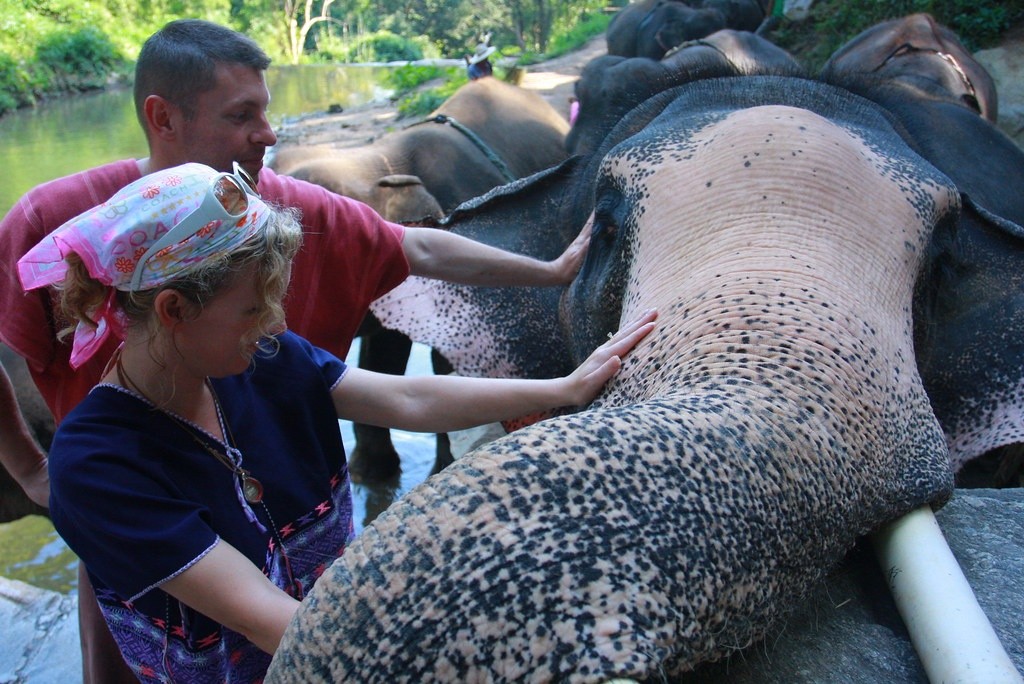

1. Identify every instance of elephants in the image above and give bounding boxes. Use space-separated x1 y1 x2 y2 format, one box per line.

263 1 1024 683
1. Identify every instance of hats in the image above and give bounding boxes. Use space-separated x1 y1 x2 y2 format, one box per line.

469 44 496 65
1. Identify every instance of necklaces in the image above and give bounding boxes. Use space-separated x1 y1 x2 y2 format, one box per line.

117 351 263 502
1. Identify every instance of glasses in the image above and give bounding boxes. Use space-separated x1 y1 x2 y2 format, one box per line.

130 160 262 291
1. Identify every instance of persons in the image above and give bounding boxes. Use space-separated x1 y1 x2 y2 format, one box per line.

467 44 496 80
17 162 657 684
0 18 597 684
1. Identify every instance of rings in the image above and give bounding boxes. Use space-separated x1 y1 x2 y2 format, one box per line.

607 332 614 339
